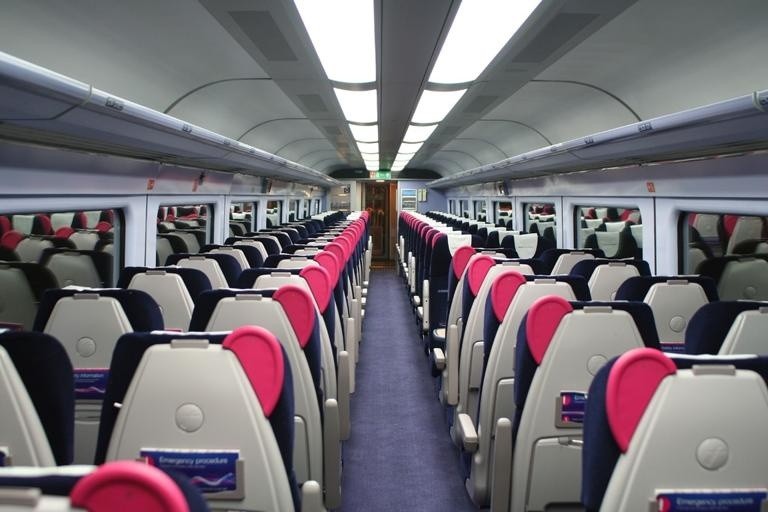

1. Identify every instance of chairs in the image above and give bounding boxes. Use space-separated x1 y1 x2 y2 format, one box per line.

0 205 373 512
395 203 768 511
